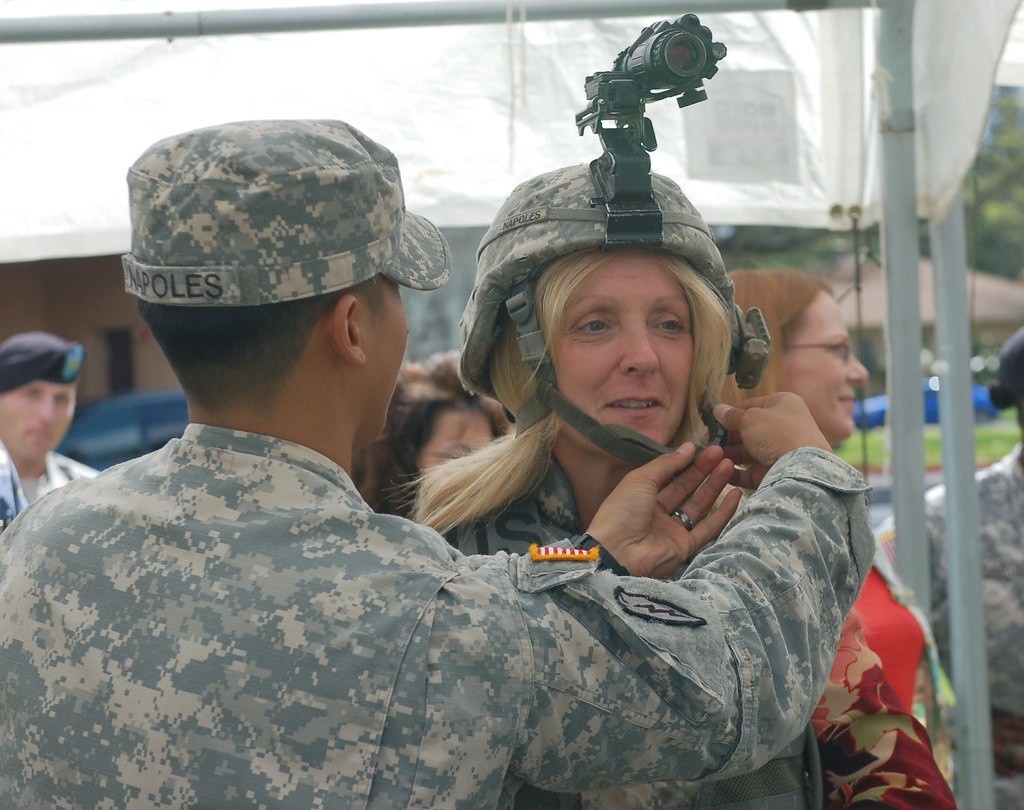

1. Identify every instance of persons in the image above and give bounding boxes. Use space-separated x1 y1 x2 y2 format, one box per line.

0 117 1023 810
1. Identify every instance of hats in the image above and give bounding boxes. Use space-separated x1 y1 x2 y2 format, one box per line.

121 119 451 306
989 328 1023 409
0 331 87 394
458 152 773 397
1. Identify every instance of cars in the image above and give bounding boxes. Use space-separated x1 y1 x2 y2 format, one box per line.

47 386 187 471
854 376 999 432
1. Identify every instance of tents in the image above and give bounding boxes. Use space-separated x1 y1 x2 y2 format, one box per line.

0 0 1024 807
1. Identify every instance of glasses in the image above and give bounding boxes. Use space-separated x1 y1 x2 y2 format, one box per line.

784 340 852 362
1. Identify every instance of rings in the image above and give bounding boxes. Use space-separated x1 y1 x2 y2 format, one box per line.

671 509 693 533
